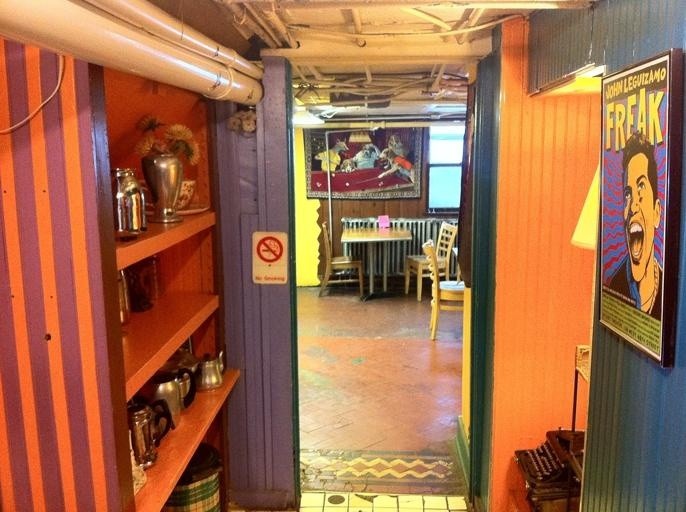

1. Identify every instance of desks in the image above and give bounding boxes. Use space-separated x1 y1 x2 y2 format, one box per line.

340 227 412 302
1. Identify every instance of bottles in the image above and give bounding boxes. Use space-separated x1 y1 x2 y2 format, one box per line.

117 269 131 327
107 166 147 242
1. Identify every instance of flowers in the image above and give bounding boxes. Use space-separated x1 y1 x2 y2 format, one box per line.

132 113 202 166
225 110 256 140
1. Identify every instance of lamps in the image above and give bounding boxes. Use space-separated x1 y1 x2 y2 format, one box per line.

569 162 602 252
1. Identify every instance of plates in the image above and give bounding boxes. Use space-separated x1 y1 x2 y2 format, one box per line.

141 205 211 216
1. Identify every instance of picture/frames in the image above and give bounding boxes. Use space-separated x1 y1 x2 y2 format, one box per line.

302 128 423 199
598 47 685 370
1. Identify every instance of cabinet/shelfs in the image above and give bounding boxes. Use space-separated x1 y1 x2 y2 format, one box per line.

114 208 242 512
545 345 592 512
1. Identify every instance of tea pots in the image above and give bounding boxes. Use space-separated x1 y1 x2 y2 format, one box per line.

126 349 225 499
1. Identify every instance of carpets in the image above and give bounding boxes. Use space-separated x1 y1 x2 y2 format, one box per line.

298 449 469 496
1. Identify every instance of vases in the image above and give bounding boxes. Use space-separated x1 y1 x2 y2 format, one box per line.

141 153 184 223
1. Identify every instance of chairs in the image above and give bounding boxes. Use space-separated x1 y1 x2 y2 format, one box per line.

319 222 364 301
405 221 458 302
420 239 465 341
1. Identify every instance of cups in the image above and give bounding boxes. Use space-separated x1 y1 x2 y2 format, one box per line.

138 179 196 210
140 252 166 300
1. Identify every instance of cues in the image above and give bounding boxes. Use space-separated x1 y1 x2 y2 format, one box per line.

356 177 378 184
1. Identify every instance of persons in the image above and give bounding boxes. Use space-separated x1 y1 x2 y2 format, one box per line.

605 131 663 320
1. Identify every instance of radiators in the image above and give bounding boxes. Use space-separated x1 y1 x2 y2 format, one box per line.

330 218 458 278
